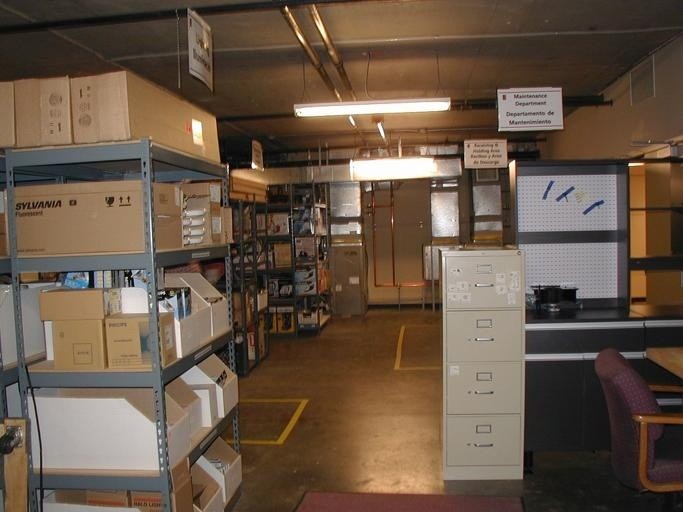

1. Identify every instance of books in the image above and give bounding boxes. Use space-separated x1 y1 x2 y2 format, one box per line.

60 267 192 321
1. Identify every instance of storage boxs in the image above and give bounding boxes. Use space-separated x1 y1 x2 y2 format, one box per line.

70 72 222 164
14 76 73 146
0 80 15 148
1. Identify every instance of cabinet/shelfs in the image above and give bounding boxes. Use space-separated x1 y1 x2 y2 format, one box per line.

438 242 533 482
528 328 647 451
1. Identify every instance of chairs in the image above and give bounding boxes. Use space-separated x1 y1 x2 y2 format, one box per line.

595 348 679 511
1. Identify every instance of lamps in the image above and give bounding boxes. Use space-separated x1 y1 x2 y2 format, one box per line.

292 44 451 119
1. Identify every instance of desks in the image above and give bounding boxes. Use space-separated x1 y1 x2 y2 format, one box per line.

640 346 682 380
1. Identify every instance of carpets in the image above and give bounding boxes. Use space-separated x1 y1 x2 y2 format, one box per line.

294 487 529 512
